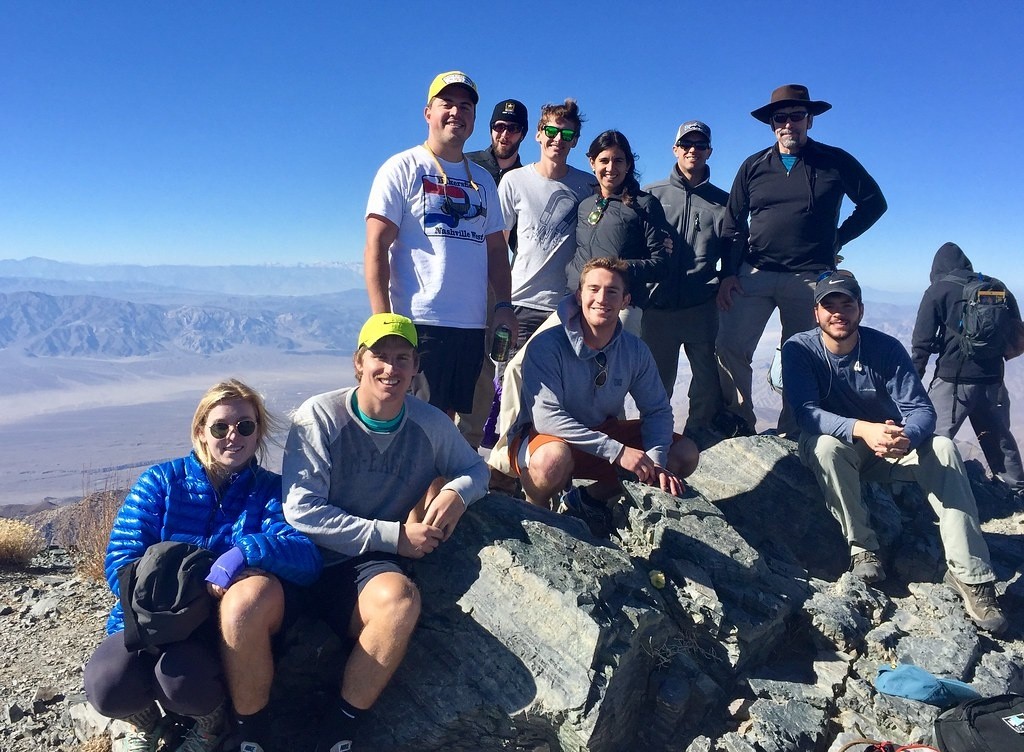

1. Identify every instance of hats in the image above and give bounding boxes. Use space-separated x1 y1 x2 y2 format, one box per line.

491 98 530 127
751 84 833 124
812 272 863 302
675 120 712 147
428 71 480 103
359 312 421 348
874 662 983 710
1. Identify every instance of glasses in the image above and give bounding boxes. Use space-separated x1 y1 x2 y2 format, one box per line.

813 268 855 282
541 126 577 143
587 198 610 226
677 140 709 150
595 350 609 387
770 111 812 123
492 123 525 134
207 419 259 440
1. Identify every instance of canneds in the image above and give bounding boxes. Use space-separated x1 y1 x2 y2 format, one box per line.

490 327 511 362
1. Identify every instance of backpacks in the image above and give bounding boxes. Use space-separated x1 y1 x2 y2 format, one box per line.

940 273 1014 361
938 689 1024 752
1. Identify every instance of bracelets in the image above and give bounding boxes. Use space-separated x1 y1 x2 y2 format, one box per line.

495 302 516 311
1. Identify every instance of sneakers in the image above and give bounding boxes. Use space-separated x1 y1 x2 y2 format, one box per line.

850 550 887 583
941 569 1009 635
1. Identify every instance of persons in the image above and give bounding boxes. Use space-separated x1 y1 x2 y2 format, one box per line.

697 85 888 447
364 71 518 435
216 312 492 752
642 117 738 448
452 99 528 453
83 377 321 752
487 256 700 519
912 243 1024 500
564 129 669 338
779 270 1008 633
496 97 672 348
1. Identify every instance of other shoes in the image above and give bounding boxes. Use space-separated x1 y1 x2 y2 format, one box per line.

314 734 353 752
178 717 231 752
122 716 166 751
225 735 265 752
